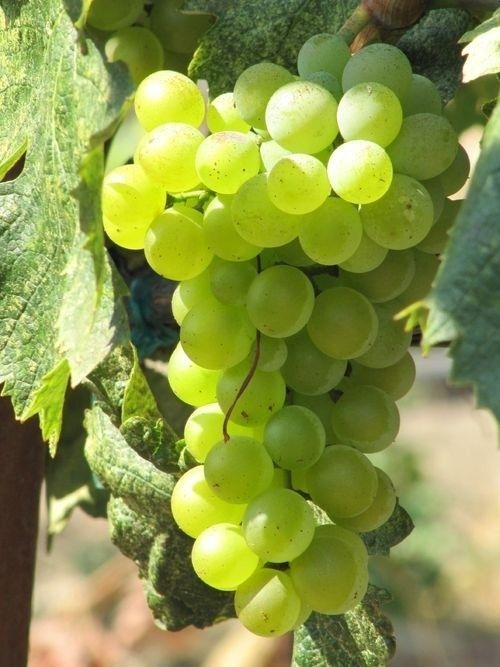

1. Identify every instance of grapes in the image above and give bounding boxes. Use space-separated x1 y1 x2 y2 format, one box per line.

100 33 469 637
86 0 216 91
